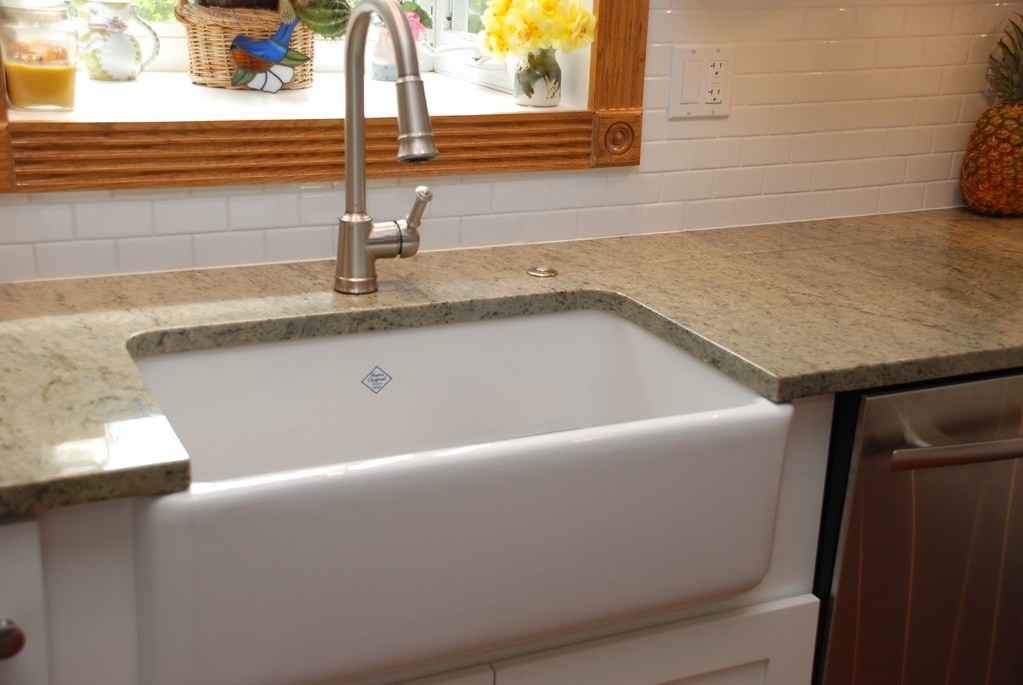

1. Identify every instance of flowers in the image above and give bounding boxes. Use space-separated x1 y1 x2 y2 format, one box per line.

382 0 432 47
482 0 597 56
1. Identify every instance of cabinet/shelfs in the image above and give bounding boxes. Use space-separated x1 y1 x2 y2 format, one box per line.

815 372 1023 685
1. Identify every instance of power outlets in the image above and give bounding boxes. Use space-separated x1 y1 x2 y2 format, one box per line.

669 44 735 118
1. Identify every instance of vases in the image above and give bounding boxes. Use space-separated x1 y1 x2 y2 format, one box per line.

369 26 400 80
514 48 561 109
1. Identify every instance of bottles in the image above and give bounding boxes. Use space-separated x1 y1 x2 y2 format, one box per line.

71 2 162 82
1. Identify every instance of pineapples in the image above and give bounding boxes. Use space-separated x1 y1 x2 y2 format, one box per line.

961 13 1023 216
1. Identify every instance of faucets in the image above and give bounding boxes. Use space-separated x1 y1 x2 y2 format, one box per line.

333 0 440 295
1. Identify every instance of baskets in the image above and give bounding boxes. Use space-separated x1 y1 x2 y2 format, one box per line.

174 0 314 90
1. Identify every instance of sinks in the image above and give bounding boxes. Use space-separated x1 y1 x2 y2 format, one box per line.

130 307 797 685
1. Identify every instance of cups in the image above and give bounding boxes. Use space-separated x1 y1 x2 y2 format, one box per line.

1 1 76 110
